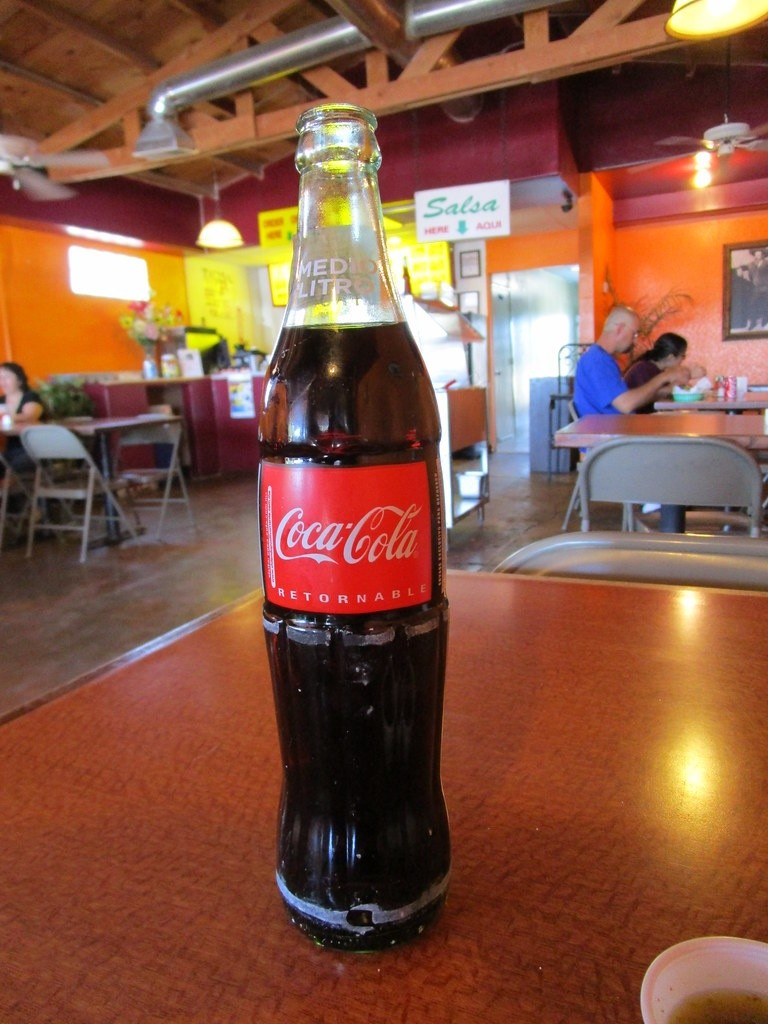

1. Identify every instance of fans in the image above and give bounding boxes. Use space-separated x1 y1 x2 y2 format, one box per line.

1 132 108 201
653 39 767 158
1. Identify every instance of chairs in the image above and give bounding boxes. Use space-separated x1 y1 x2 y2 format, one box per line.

110 418 195 540
19 423 138 563
489 439 768 591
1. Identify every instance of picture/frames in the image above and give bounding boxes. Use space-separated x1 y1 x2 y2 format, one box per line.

722 239 768 340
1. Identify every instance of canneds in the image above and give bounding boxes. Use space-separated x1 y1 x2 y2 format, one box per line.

725 375 737 398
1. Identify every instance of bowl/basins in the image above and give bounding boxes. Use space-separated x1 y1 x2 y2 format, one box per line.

641 936 767 1024
674 393 704 401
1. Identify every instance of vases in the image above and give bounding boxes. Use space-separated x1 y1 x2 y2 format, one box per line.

144 350 161 382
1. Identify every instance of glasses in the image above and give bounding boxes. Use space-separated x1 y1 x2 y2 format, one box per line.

616 322 640 337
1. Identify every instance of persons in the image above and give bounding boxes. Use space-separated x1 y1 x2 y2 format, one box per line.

624 333 706 415
0 362 51 482
574 305 691 515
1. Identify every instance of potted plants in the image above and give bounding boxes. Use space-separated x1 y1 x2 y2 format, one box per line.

45 380 93 425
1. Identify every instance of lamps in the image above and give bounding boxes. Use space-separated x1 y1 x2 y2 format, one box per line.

195 170 246 249
664 0 768 43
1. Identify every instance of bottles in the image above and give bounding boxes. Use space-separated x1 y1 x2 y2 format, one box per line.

713 376 727 397
257 105 456 950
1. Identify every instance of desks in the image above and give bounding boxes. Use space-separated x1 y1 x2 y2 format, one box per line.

1 413 171 547
429 387 491 548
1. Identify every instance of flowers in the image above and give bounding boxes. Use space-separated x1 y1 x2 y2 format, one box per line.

119 299 192 357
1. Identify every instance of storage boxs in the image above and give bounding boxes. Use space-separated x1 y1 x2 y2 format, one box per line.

455 470 487 498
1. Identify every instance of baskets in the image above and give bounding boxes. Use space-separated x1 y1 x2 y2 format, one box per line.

673 393 704 402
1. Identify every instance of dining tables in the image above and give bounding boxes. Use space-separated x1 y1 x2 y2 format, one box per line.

556 413 768 530
654 391 768 415
2 571 768 1022
